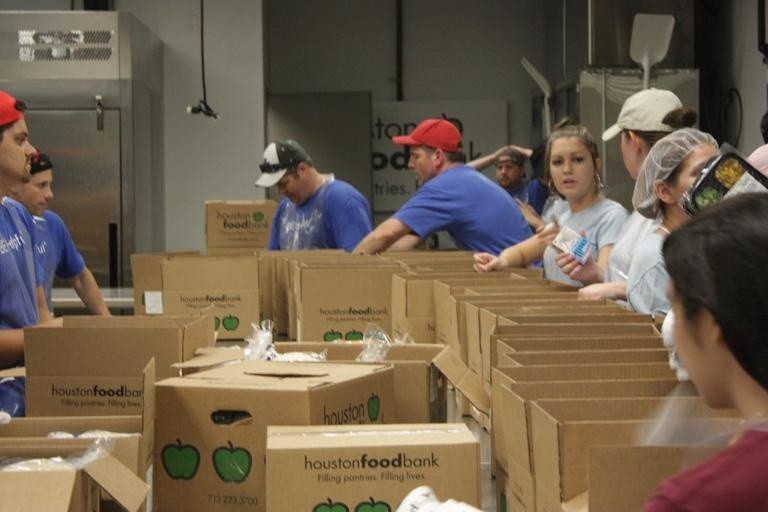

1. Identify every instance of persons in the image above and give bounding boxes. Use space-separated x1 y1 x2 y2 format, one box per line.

524 141 551 214
466 143 535 204
626 127 722 315
642 192 768 512
14 154 115 317
554 86 698 302
0 90 63 422
350 117 547 268
472 115 630 286
254 139 371 252
746 109 768 178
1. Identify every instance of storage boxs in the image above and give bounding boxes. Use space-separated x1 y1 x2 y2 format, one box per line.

434 281 648 429
163 259 262 340
206 203 276 247
197 341 490 422
490 365 681 456
155 358 396 510
266 422 482 512
379 245 525 283
129 255 164 315
391 274 525 351
526 394 699 512
24 314 198 422
0 443 150 511
500 383 702 511
257 243 404 344
4 357 153 511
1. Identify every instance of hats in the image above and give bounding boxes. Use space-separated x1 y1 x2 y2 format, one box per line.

602 88 689 143
632 127 717 219
392 118 464 154
495 149 525 166
256 141 301 189
530 142 546 180
0 89 24 128
31 151 53 174
